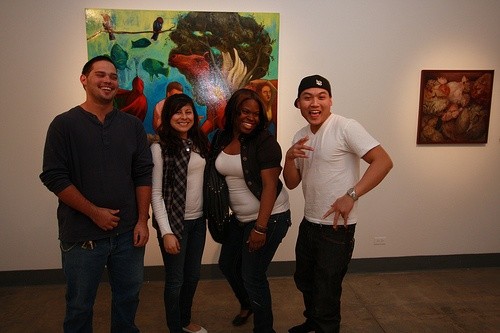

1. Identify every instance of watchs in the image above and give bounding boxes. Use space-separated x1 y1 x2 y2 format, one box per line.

347 188 358 201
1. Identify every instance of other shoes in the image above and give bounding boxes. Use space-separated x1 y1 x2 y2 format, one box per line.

289 319 315 333
183 323 207 333
232 307 253 326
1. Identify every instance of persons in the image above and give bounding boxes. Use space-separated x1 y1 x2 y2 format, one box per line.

149 94 210 333
39 55 154 333
283 75 393 333
203 88 292 333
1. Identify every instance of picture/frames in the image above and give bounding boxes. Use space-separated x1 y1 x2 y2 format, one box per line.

416 69 494 145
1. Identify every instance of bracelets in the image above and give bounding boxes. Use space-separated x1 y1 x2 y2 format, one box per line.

253 225 267 235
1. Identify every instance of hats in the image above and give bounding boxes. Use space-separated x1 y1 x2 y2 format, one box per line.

295 75 331 108
